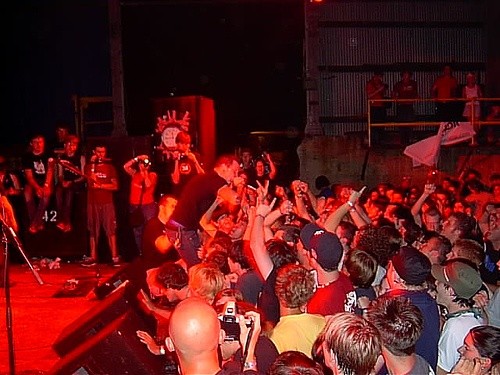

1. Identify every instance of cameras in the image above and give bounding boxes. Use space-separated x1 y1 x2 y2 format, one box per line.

95 157 103 163
218 300 254 324
143 159 151 167
179 152 187 159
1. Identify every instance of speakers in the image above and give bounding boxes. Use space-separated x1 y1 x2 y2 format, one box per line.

52 265 171 375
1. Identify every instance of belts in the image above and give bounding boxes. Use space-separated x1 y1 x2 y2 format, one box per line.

165 223 197 232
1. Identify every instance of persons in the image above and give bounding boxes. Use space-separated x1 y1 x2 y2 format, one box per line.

0 126 500 375
433 65 461 120
367 69 389 143
391 69 418 143
461 70 483 121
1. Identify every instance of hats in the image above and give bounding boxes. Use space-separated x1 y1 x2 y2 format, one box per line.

431 262 482 299
156 263 189 290
389 243 431 284
300 223 343 267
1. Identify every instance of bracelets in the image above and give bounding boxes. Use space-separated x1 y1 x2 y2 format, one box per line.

362 308 368 310
255 214 265 221
348 201 353 206
279 209 283 214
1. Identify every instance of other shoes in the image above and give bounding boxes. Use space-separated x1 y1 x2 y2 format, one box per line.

37 224 44 230
29 225 38 234
80 257 97 266
64 224 71 232
57 222 65 231
111 257 121 266
